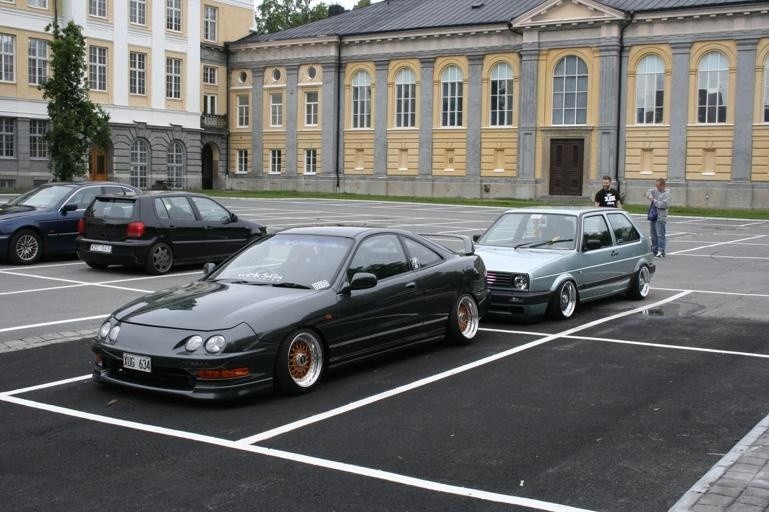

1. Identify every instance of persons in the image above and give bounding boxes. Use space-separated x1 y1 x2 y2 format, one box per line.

643 178 672 259
594 176 621 208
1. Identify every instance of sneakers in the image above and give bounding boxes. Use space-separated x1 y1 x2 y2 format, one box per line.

653 250 666 258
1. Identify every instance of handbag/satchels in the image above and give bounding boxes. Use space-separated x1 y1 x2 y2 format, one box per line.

647 200 659 223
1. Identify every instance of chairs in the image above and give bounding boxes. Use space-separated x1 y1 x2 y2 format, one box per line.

276 244 349 290
107 205 126 218
160 206 193 219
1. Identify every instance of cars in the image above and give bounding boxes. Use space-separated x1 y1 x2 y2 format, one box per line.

472 205 659 329
91 223 489 409
76 187 269 277
0 178 149 268
